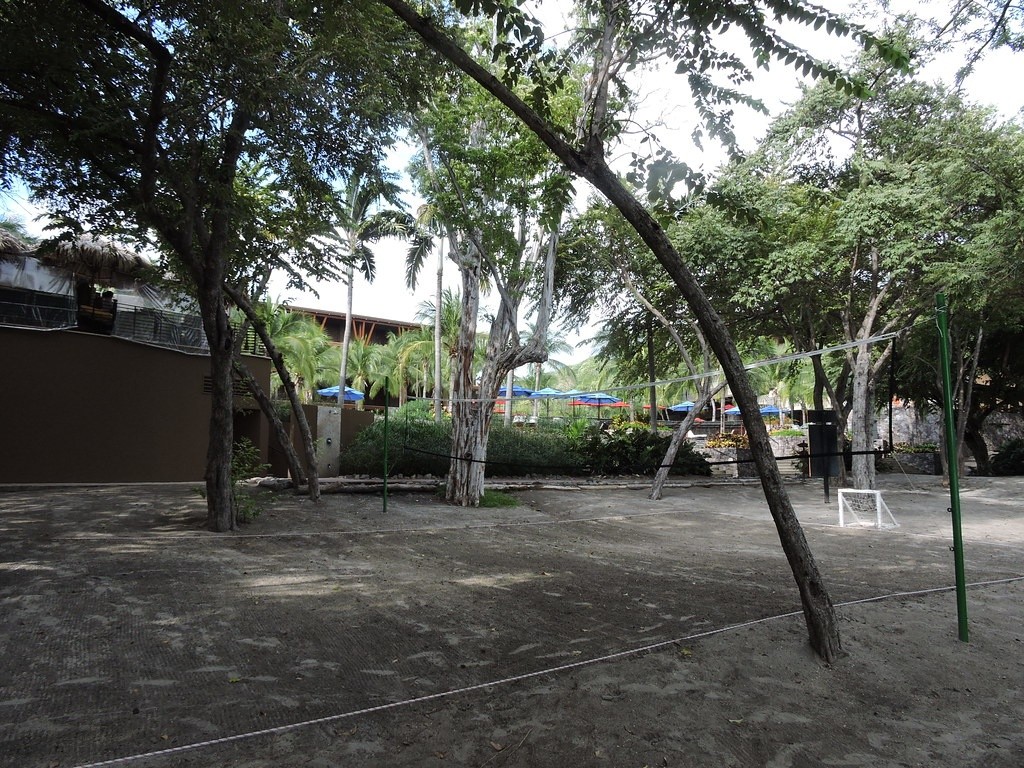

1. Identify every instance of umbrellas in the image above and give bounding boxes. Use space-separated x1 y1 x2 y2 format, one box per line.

716 404 787 435
670 400 708 412
37 233 162 291
641 405 667 410
493 385 629 418
694 417 704 422
316 385 364 402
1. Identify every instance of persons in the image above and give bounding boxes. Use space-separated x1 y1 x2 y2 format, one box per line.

100 291 115 311
76 276 91 311
